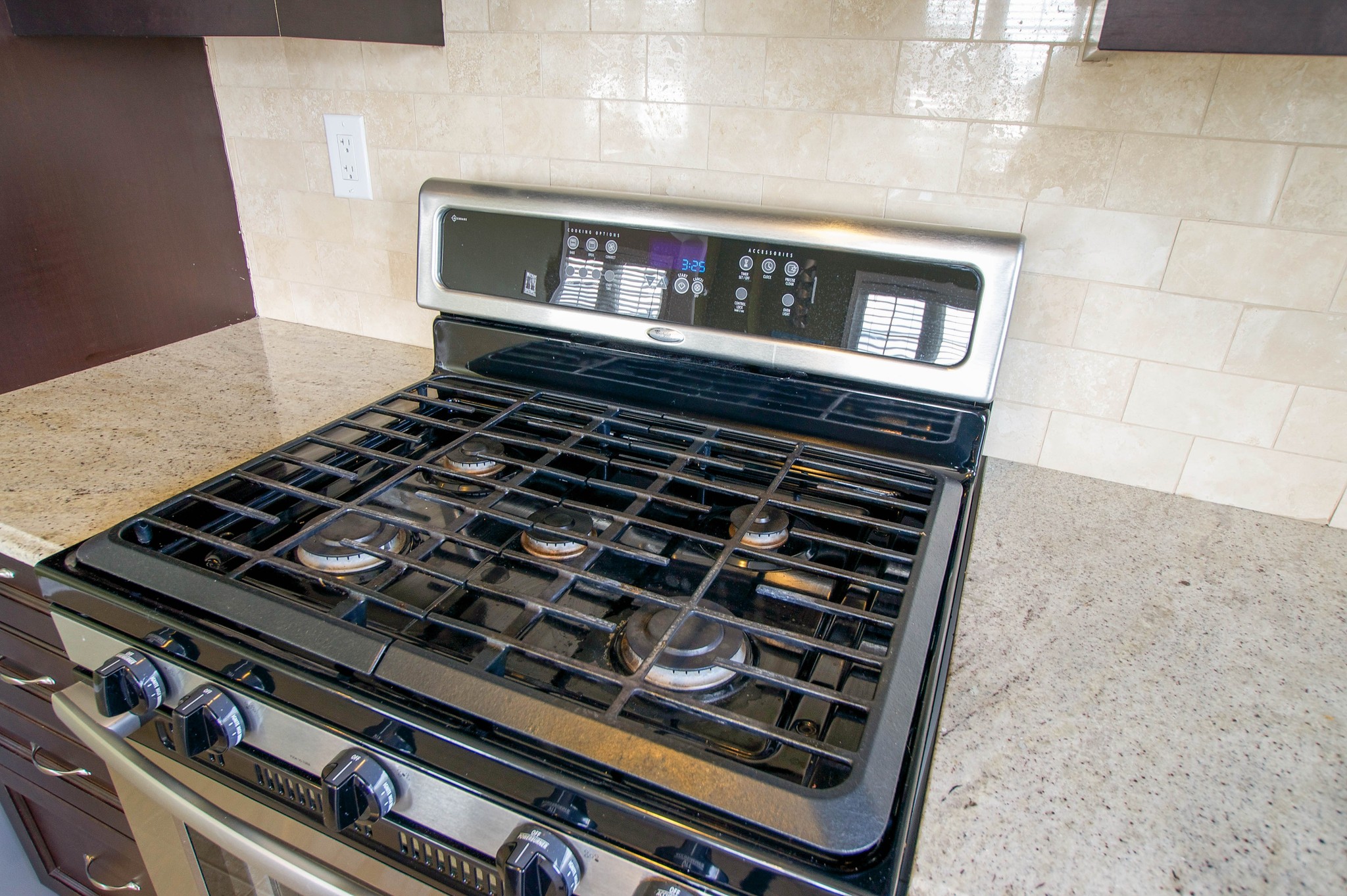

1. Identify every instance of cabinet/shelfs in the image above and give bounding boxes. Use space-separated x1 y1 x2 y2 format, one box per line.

0 526 157 896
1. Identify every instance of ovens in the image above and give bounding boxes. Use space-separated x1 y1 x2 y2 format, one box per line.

44 677 457 896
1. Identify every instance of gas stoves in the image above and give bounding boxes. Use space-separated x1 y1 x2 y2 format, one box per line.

31 174 1026 896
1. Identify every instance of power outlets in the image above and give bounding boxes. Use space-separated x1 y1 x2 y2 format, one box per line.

324 113 374 199
